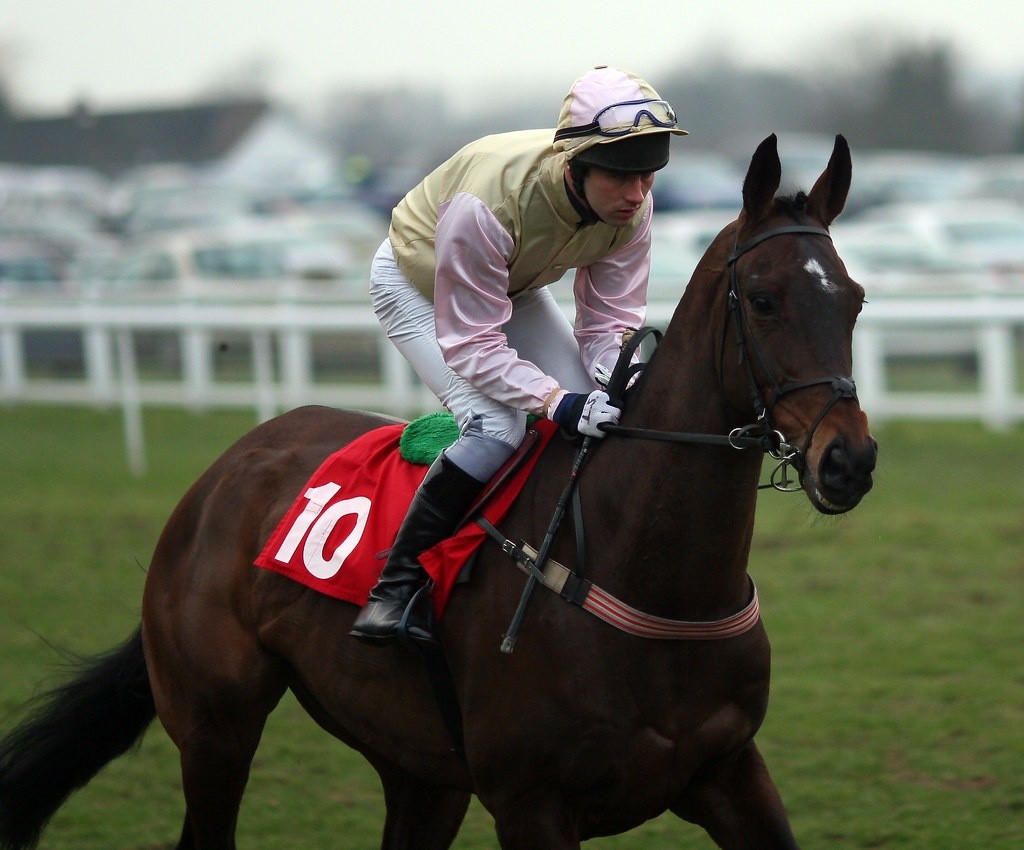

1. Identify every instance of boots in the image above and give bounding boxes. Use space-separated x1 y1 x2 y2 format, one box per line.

348 448 487 657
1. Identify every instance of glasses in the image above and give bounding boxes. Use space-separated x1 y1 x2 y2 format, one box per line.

553 97 678 144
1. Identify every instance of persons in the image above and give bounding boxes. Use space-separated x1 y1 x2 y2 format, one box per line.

348 66 689 651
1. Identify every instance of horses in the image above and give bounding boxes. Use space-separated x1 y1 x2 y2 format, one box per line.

1 133 879 850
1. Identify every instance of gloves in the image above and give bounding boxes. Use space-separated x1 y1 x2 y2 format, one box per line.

595 363 642 389
553 389 622 440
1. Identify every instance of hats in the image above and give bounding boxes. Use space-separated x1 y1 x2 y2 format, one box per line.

572 131 670 176
